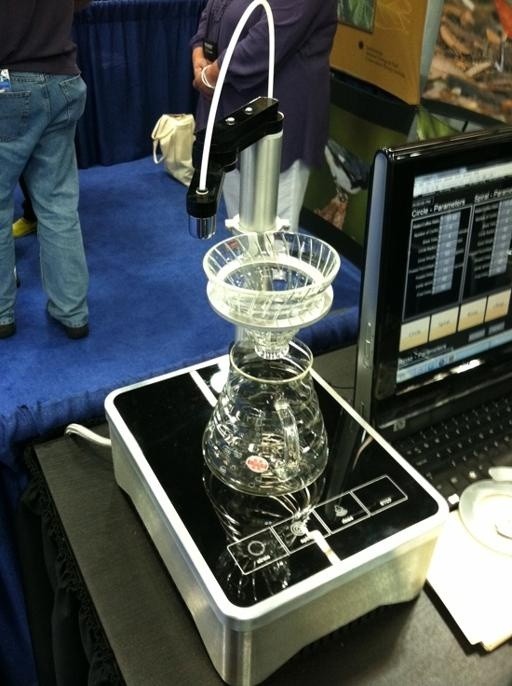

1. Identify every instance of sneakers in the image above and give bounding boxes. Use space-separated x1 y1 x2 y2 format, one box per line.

0 315 20 341
11 214 37 239
43 300 91 341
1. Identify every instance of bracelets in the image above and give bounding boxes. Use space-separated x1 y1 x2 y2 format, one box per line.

200 64 216 90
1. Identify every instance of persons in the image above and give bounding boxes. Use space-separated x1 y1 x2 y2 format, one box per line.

187 1 340 235
0 0 97 340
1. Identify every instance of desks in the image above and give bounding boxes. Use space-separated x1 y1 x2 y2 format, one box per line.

32 348 512 686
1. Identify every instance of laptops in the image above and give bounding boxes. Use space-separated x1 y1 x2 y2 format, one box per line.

354 125 512 652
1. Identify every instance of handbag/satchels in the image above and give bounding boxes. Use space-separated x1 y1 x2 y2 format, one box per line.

147 109 199 189
